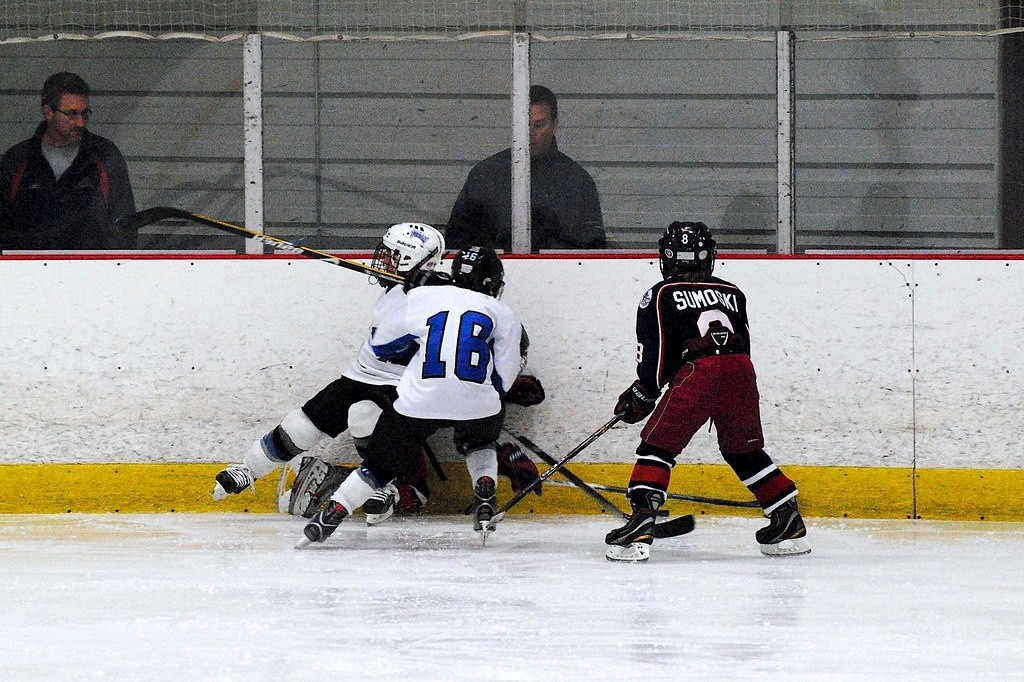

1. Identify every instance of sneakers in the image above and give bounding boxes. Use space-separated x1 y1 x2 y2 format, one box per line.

755 495 812 556
278 450 330 519
212 463 259 500
605 487 667 563
361 476 401 527
470 477 496 547
294 500 349 550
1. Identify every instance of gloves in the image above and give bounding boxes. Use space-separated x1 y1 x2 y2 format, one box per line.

504 375 545 408
495 441 543 497
614 379 662 424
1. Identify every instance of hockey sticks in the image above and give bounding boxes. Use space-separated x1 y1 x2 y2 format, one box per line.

501 420 696 538
538 477 766 509
114 204 406 285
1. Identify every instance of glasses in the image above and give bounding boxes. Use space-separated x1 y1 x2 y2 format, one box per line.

47 103 93 119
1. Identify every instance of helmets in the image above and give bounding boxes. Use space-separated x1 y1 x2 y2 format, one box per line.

488 323 530 368
450 246 504 298
658 222 716 279
378 222 445 287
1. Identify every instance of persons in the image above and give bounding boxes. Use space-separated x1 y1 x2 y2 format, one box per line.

210 223 544 547
0 71 136 250
604 221 813 562
445 84 607 247
